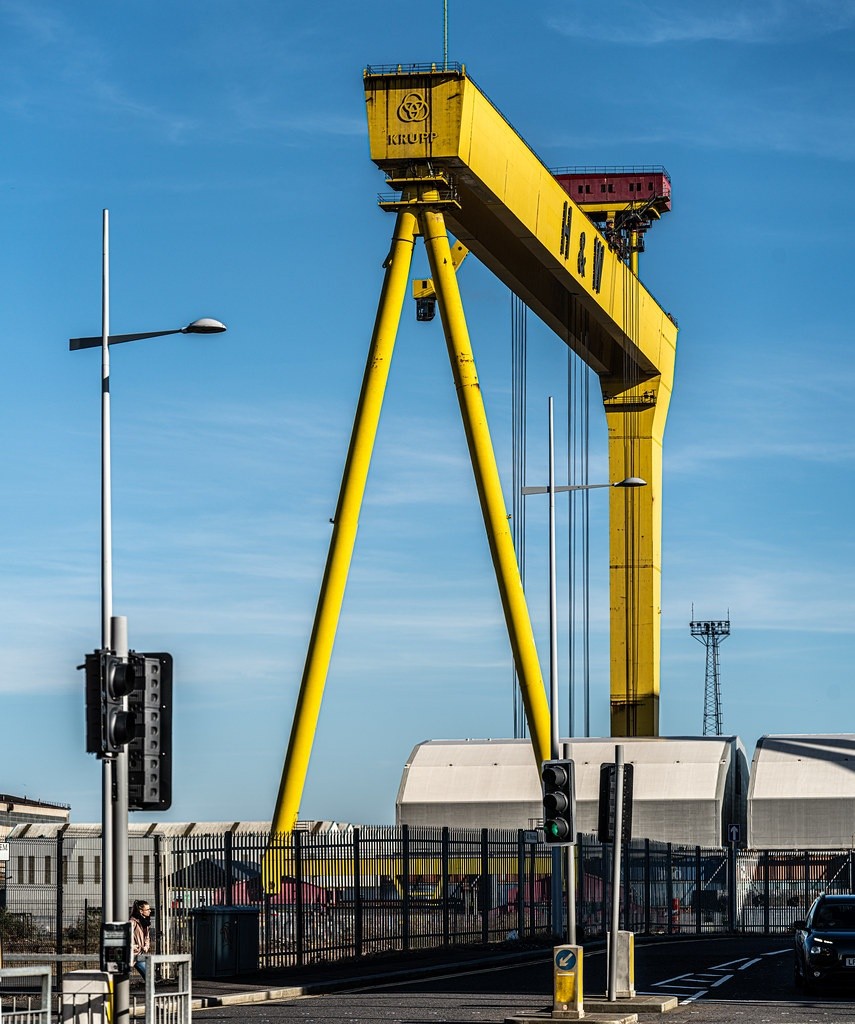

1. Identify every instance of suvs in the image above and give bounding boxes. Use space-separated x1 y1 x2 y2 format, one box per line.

792 893 855 985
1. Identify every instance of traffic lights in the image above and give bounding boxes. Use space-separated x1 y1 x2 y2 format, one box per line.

540 759 577 849
74 648 107 759
105 654 138 756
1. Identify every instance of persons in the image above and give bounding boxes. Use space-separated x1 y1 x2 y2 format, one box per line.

129 899 168 1016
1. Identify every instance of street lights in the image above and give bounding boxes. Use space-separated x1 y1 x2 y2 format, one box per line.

64 208 227 1023
